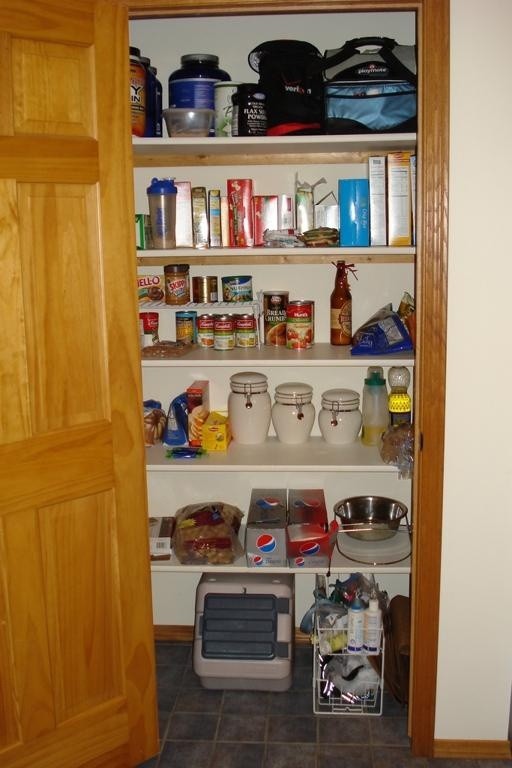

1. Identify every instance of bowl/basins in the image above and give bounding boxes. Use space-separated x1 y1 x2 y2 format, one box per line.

332 496 407 542
161 107 217 135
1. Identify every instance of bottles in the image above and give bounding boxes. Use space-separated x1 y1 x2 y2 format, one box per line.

346 597 364 655
329 259 352 343
361 369 389 445
145 178 179 250
233 83 266 135
130 46 162 135
363 599 382 655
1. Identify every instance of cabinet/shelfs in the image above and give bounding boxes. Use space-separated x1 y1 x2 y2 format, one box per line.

132 133 418 577
312 574 387 718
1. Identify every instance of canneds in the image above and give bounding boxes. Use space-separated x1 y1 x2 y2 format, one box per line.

193 276 218 303
140 312 160 349
222 276 253 302
164 264 191 305
176 311 258 350
263 291 315 351
213 81 243 136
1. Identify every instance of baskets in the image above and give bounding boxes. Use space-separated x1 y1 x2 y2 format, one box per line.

312 611 384 715
